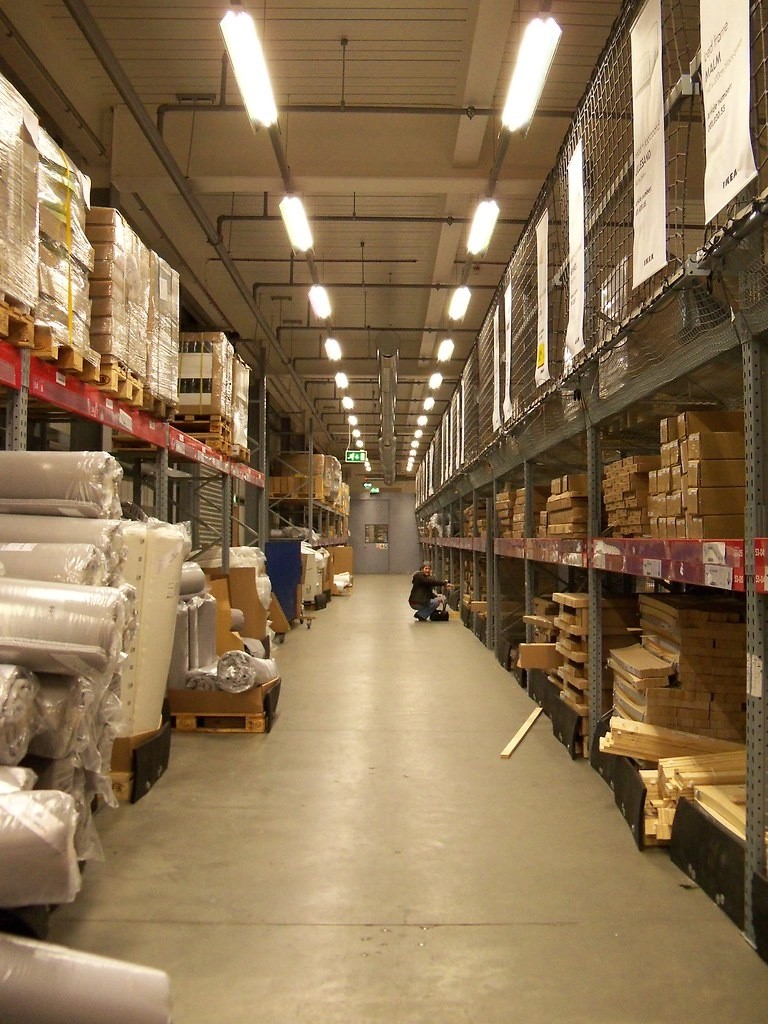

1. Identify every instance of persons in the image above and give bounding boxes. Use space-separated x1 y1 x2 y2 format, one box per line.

408 561 448 621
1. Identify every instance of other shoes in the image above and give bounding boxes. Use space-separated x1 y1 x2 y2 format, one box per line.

414 612 426 621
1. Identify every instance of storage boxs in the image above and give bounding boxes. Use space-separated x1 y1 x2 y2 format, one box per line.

180 326 231 419
97 711 163 769
1 74 183 414
169 672 280 713
229 350 252 447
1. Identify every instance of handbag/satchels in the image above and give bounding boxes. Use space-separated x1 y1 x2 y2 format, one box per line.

430 609 450 621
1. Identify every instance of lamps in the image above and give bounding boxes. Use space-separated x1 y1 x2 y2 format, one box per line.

216 11 565 472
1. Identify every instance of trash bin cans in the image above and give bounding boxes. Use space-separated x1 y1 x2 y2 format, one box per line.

265 538 304 620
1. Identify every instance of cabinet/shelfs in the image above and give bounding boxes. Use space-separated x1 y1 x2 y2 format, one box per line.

267 477 493 656
487 452 529 699
597 331 767 956
524 419 595 771
